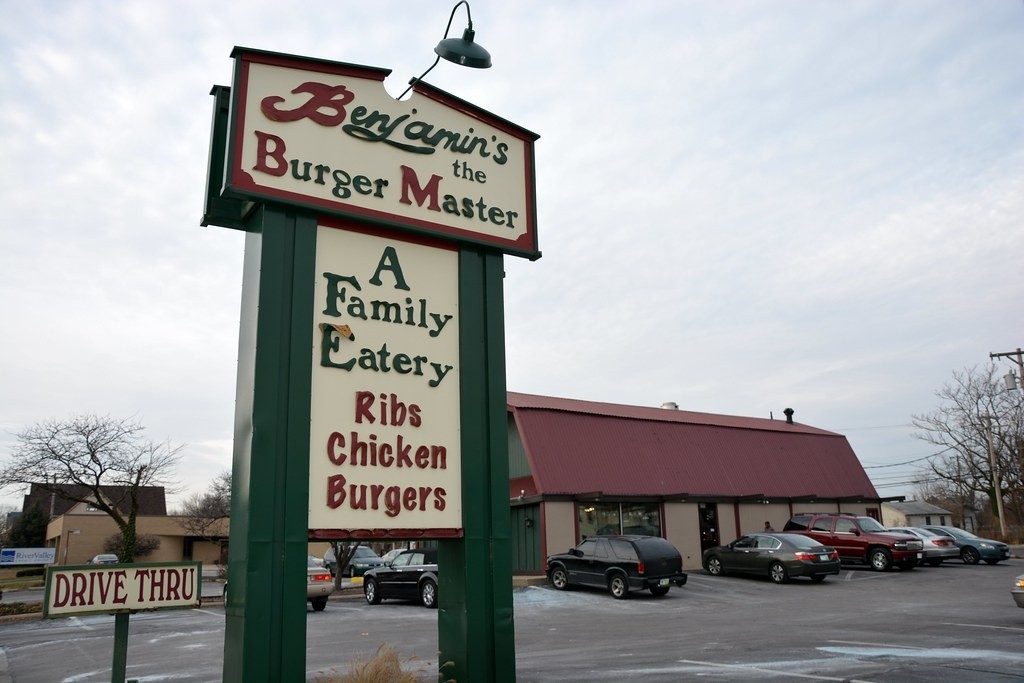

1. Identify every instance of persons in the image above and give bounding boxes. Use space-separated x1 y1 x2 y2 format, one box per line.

763 520 777 535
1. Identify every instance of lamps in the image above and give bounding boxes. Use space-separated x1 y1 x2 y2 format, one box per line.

397 1 491 104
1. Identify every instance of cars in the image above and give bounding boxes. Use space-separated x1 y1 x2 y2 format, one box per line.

380 547 425 567
885 526 960 568
363 548 439 609
921 525 1010 566
221 555 334 612
86 554 120 566
322 543 386 577
702 534 841 583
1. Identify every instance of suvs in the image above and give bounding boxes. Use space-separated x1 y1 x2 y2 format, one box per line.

783 511 925 573
544 534 688 599
